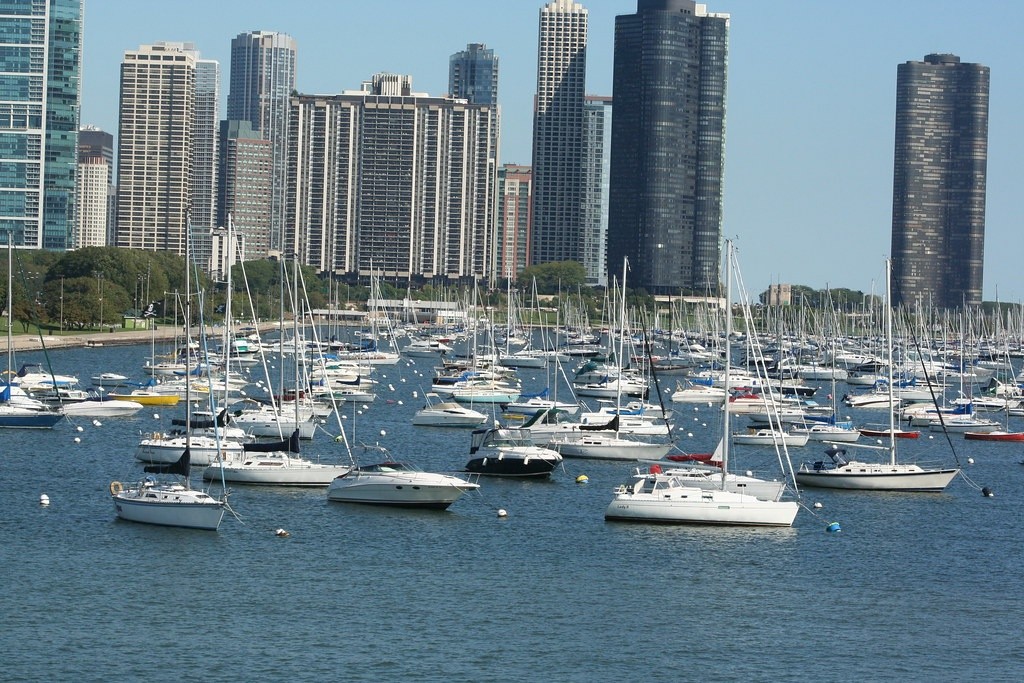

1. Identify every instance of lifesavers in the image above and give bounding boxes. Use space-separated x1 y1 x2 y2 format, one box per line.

110 481 123 495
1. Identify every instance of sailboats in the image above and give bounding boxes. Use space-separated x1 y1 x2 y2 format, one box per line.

792 258 962 491
294 255 482 507
0 212 1023 482
603 237 803 530
109 288 234 531
